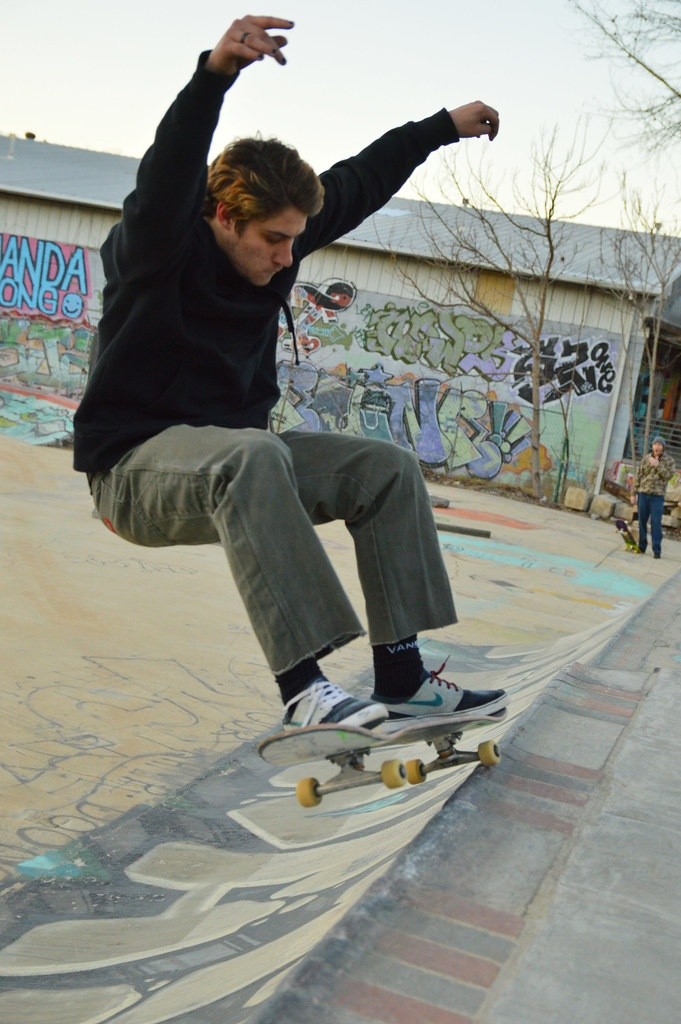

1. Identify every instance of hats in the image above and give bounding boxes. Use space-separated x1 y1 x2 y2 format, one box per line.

652 437 666 446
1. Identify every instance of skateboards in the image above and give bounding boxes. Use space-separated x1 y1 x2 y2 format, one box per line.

614 520 641 554
255 706 509 809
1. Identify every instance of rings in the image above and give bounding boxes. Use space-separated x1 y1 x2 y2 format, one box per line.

240 31 250 44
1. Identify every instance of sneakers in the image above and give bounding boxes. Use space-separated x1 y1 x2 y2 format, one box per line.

371 654 508 723
281 675 389 729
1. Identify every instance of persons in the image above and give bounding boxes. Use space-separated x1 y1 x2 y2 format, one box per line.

630 436 676 559
72 15 512 733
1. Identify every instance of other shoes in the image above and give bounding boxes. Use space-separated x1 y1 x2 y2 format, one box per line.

639 547 645 553
654 553 660 558
